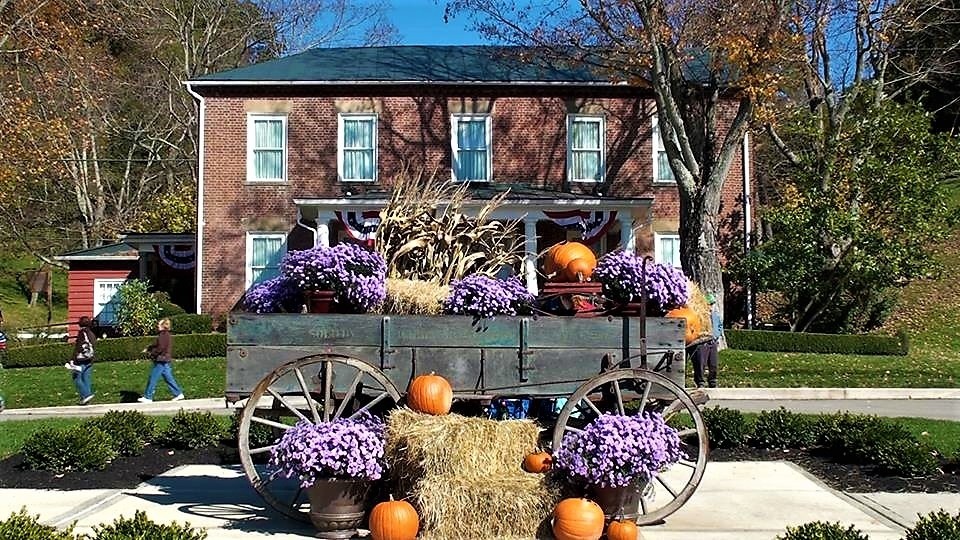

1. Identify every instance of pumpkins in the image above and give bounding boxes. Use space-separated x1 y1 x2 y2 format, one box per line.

524 447 552 473
408 371 453 415
607 515 638 540
544 241 597 283
369 493 420 540
550 497 604 540
662 308 702 344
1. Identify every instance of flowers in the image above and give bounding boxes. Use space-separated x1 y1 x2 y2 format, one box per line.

551 410 689 502
262 407 393 489
441 273 535 321
242 243 387 314
596 248 687 318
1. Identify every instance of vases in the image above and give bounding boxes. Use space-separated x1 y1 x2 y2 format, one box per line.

306 290 334 313
569 476 645 527
617 302 643 318
307 478 368 538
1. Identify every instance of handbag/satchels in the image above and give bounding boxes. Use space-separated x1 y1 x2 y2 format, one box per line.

74 330 93 365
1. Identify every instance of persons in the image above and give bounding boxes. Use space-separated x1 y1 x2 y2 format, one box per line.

687 294 723 388
136 317 184 402
71 316 96 405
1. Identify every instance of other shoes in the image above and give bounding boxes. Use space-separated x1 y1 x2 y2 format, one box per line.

171 394 184 401
80 394 95 405
138 397 151 403
